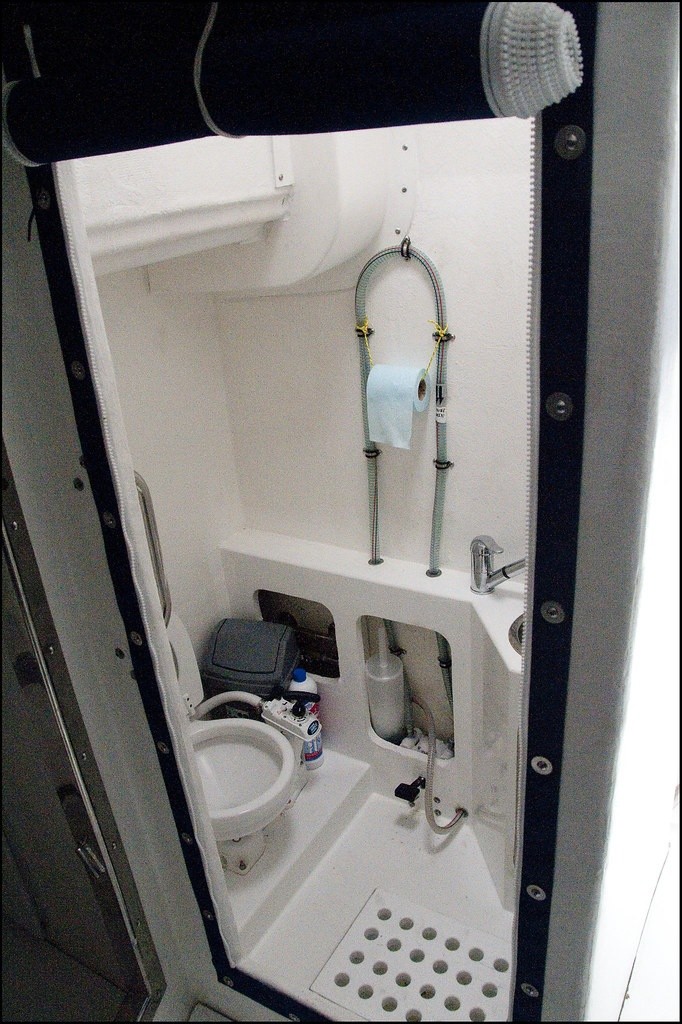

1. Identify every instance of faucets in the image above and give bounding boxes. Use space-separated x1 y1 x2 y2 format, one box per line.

470 531 529 596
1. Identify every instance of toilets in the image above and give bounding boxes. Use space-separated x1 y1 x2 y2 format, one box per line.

156 608 298 878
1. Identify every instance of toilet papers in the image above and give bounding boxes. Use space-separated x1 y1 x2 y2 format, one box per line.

365 361 432 451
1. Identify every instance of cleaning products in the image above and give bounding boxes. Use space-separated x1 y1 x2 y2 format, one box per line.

289 661 323 735
279 687 327 767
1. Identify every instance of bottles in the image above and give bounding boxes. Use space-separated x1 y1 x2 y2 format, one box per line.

367 623 407 746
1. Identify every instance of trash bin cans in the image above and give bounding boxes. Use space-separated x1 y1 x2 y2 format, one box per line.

201 616 301 723
